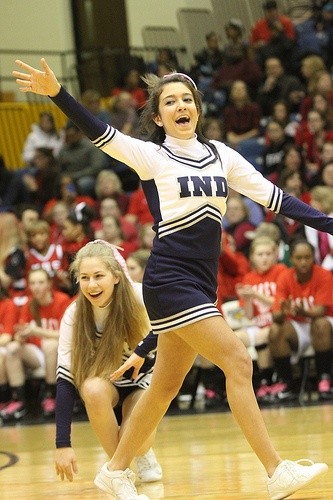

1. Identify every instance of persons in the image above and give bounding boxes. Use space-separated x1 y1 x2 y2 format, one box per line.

54 241 163 484
10 59 333 500
0 0 333 423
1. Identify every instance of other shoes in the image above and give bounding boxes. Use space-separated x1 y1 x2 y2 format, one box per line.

255 385 271 401
204 389 219 409
318 377 333 399
43 397 56 417
0 400 29 420
272 382 295 400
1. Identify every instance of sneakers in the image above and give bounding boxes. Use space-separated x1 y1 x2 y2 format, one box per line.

95 465 151 500
266 458 328 500
135 450 161 482
